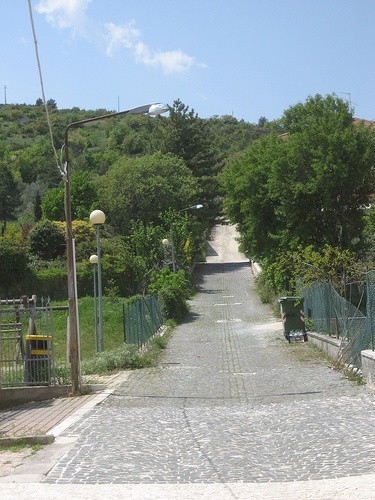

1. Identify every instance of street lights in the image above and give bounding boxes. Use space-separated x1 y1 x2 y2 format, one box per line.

62 102 169 401
161 238 170 272
89 209 107 355
89 255 99 357
171 204 203 274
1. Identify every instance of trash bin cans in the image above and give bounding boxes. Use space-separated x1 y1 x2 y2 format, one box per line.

278 296 308 342
23 334 51 386
